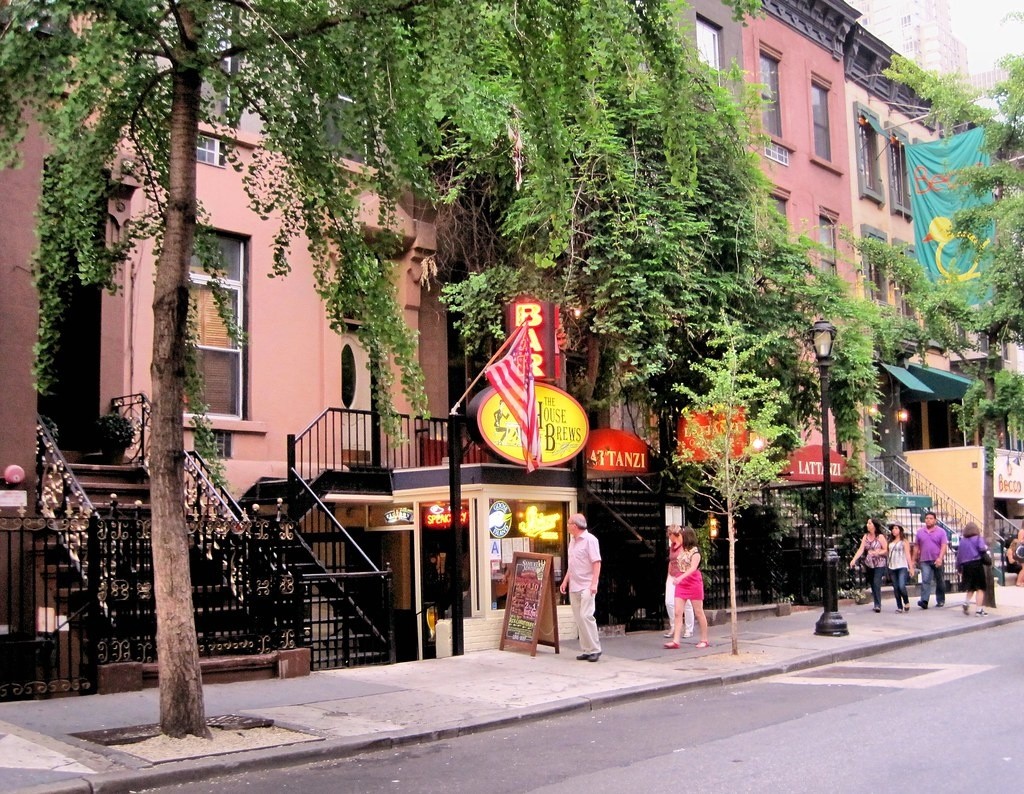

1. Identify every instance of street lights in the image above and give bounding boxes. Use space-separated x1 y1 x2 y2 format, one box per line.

802 317 851 637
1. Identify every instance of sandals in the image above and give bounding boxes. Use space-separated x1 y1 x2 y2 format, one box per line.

577 653 589 660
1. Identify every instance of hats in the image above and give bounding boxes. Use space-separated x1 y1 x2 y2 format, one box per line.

888 521 904 532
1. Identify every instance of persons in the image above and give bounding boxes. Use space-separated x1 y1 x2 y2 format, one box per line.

955 521 994 617
911 512 948 609
664 525 709 648
1016 520 1024 587
665 526 695 638
850 518 890 613
874 520 915 614
559 511 603 662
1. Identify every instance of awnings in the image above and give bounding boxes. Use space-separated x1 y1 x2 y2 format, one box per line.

905 360 988 400
878 361 934 394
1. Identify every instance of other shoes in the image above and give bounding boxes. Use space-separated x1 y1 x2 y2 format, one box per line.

665 632 674 638
918 601 928 609
896 608 903 613
589 651 599 661
873 607 882 612
682 633 693 638
904 602 909 611
664 641 678 649
963 604 969 615
936 602 943 608
975 610 987 617
696 641 709 648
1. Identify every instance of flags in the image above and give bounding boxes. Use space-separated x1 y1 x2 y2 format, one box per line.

486 323 543 473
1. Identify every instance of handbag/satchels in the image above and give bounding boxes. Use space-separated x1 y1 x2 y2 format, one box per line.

976 536 992 567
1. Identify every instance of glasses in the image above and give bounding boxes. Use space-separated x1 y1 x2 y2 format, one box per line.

567 522 574 526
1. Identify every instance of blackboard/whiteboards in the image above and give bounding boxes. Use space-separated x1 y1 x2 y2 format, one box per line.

501 551 559 649
